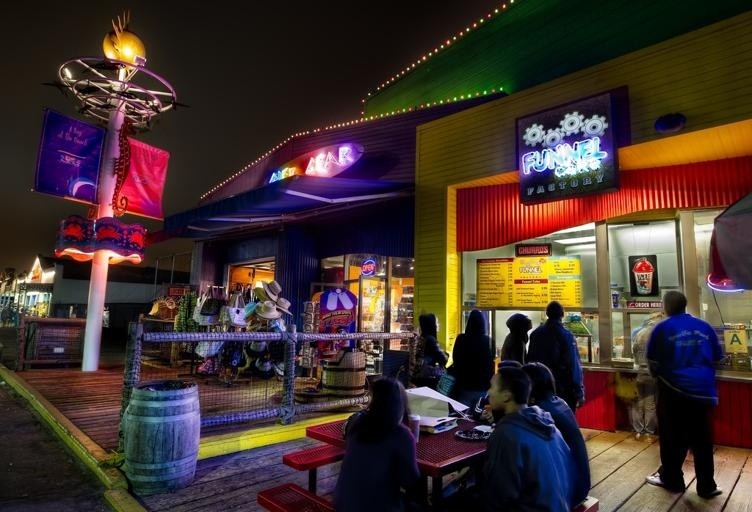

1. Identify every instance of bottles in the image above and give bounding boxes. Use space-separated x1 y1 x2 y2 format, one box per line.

632 257 655 295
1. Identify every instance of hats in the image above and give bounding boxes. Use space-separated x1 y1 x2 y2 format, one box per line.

243 279 298 382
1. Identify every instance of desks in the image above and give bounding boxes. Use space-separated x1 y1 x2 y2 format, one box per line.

306 420 487 512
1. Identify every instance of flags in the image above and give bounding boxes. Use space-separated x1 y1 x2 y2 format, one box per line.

32 106 107 206
117 134 171 222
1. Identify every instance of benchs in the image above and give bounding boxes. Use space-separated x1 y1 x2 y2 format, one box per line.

257 443 347 512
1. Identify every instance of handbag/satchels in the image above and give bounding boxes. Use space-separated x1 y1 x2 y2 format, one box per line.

193 280 248 385
405 386 471 435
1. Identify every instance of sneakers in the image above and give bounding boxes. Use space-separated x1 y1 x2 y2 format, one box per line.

646 475 686 489
701 487 722 497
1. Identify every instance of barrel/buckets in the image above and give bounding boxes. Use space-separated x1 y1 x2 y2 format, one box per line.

124 376 201 496
321 346 366 397
295 387 328 405
383 349 410 387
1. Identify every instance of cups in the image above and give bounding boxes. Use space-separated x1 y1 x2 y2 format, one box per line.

407 413 421 445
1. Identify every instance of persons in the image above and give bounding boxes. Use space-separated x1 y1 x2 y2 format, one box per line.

452 307 495 414
645 290 725 500
517 361 593 510
442 362 580 512
500 313 533 365
415 312 448 388
330 373 431 510
528 302 586 414
627 311 668 437
